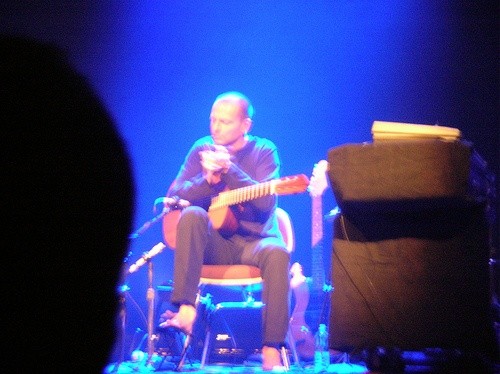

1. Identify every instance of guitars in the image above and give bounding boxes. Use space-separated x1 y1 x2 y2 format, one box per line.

162 174 310 250
288 160 341 358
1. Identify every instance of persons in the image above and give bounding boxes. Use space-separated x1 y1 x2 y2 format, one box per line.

155 92 290 372
0 20 136 374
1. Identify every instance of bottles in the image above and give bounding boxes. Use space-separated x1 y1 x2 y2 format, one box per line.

314 324 330 374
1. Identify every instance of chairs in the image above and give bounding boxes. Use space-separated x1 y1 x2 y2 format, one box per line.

175 207 301 370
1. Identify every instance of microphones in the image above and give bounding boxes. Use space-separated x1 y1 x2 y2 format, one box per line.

155 196 191 210
128 241 166 274
